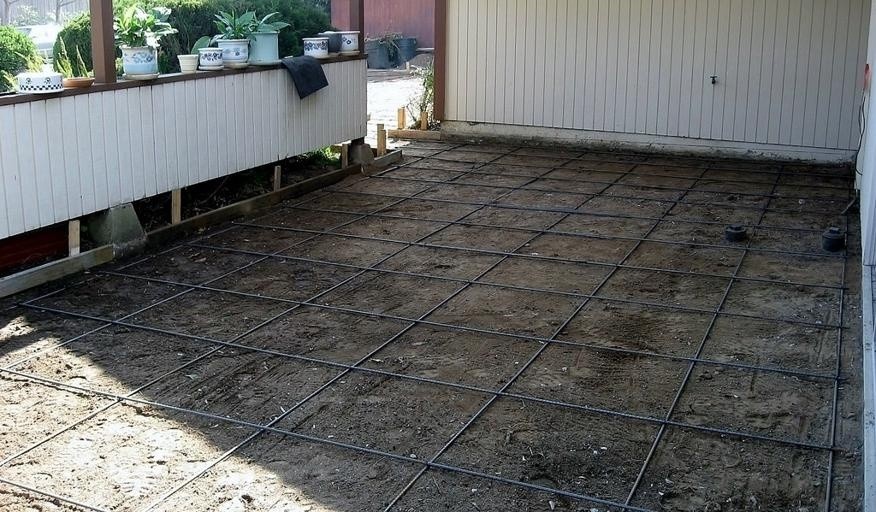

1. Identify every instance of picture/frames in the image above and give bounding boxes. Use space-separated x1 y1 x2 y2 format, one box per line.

302 37 329 58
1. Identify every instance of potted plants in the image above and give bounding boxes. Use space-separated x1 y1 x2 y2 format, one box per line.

117 3 176 80
213 8 257 68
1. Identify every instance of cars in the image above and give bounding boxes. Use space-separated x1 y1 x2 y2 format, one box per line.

14 25 65 58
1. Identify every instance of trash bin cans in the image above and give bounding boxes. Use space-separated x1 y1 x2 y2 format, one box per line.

367 37 418 69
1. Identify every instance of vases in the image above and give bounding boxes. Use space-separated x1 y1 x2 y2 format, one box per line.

318 31 342 53
63 77 96 89
198 48 225 71
177 54 199 74
249 31 279 62
334 31 360 53
18 72 65 94
